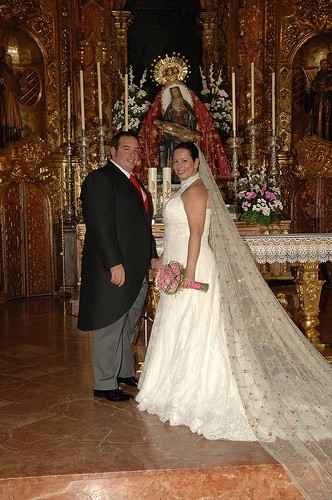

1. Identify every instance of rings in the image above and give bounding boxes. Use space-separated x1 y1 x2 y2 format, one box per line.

116 276 118 278
188 282 191 284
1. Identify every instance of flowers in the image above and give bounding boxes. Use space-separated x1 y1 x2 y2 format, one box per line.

155 260 210 298
110 64 153 135
199 61 233 142
232 158 284 228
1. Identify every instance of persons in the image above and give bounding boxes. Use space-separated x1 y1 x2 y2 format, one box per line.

0 54 23 142
305 59 332 140
77 131 159 402
160 87 196 151
135 141 332 500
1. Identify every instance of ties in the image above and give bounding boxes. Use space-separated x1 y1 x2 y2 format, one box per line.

130 174 149 217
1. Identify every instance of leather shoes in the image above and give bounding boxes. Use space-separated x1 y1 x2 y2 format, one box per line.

117 378 138 386
94 388 134 402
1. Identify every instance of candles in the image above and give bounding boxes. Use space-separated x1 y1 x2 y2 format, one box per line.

125 74 128 131
97 62 102 120
272 72 276 130
162 166 172 222
79 69 85 130
148 167 157 215
67 87 70 141
250 62 255 119
232 72 236 136
